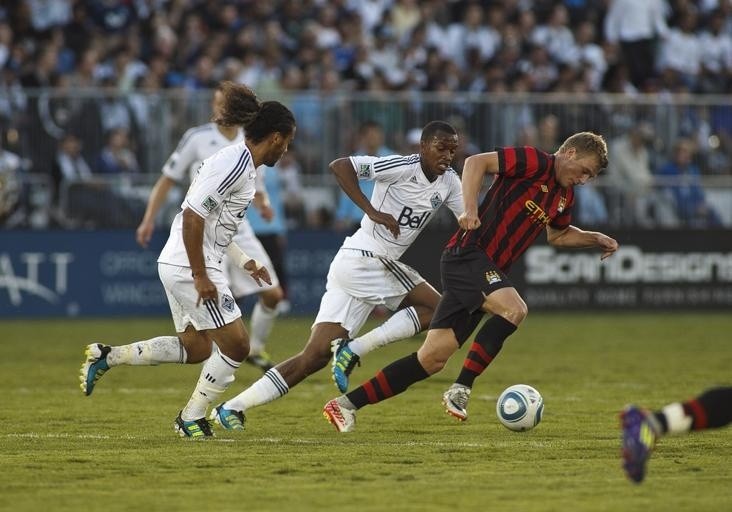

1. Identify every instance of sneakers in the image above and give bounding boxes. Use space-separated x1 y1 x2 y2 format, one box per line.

209 401 245 429
175 409 214 437
323 398 357 433
442 387 471 421
330 338 360 393
246 351 277 373
80 343 111 396
619 407 663 483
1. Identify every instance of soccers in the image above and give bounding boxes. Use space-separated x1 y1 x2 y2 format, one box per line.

496 384 544 432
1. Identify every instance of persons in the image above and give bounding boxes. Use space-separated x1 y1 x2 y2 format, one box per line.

617 384 732 484
2 2 731 228
133 80 284 374
322 130 619 431
78 99 298 438
208 119 466 429
234 166 291 317
